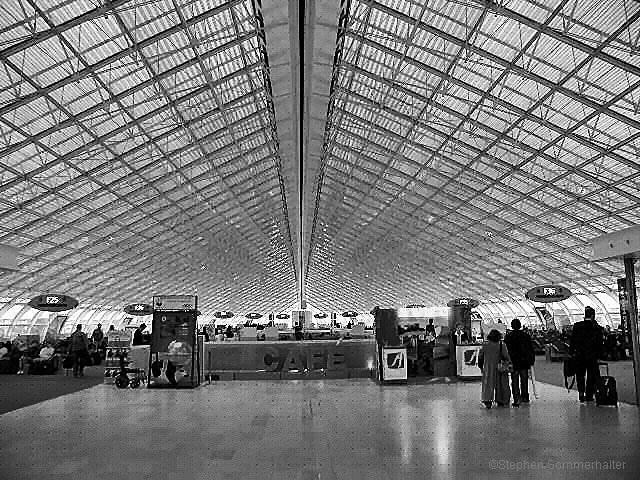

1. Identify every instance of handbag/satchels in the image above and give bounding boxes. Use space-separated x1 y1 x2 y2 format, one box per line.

497 340 514 374
80 352 92 366
564 356 577 389
63 354 74 369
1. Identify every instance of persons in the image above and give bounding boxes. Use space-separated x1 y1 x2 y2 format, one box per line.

0 341 8 358
16 339 38 374
477 330 512 409
569 306 601 403
33 342 54 363
504 319 535 407
67 324 89 377
91 320 624 352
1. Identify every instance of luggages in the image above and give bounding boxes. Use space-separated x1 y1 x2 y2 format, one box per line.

594 363 618 408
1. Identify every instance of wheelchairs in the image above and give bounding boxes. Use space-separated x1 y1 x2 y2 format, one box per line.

110 348 148 390
